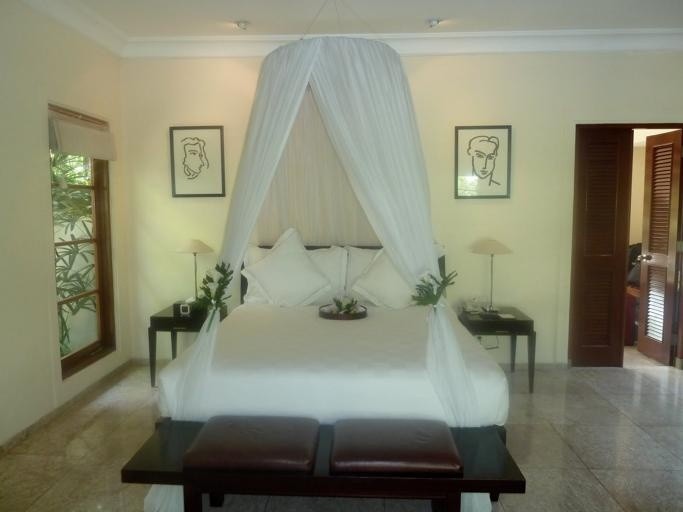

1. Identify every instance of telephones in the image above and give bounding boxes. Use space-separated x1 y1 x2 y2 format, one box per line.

462 300 483 315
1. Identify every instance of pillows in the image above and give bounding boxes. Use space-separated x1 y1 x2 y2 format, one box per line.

238 227 416 310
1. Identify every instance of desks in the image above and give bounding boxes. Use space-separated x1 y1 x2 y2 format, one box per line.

146 301 226 387
458 305 536 394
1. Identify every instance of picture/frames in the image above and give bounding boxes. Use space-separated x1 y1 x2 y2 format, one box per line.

454 125 510 200
168 125 226 199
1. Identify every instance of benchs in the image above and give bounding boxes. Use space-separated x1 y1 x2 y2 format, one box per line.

120 416 525 512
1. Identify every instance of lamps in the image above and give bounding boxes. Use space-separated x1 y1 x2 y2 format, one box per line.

174 239 215 303
469 239 513 312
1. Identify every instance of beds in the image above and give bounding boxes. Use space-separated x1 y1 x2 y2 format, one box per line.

160 243 511 504
